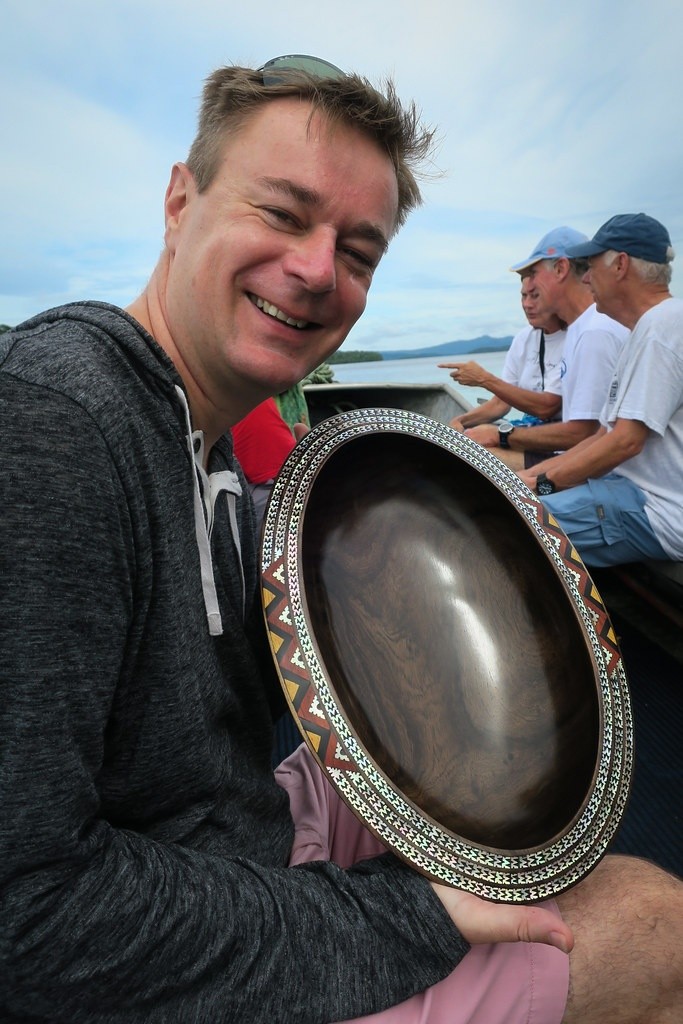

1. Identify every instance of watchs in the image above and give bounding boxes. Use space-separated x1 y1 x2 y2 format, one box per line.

537 473 554 495
498 423 514 449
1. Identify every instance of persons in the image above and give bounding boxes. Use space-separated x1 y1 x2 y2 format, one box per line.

229 396 309 549
0 65 683 1024
437 213 683 573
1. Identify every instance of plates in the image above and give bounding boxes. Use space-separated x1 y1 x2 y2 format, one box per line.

257 408 634 903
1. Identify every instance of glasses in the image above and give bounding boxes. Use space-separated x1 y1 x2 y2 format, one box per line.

255 55 347 88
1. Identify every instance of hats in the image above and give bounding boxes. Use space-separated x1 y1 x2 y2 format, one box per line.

511 226 589 274
565 213 673 264
